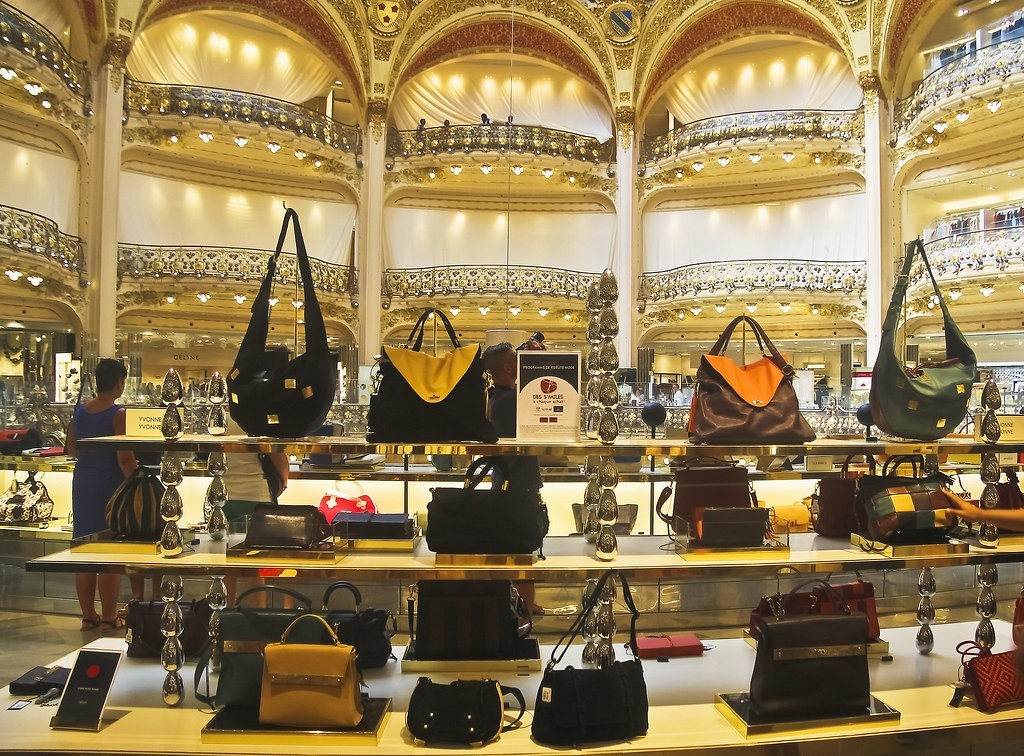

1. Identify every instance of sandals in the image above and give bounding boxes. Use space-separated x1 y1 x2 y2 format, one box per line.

80 615 102 631
101 616 126 633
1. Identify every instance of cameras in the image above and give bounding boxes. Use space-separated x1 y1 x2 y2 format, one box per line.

515 331 545 352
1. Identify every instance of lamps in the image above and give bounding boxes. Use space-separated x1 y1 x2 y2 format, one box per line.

1 61 1024 317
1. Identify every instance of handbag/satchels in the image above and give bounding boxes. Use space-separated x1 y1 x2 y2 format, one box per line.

0 206 1024 756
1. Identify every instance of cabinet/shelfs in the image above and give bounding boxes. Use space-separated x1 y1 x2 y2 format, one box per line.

0 436 1024 756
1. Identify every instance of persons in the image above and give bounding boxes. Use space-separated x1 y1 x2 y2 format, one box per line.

671 384 685 406
505 115 513 138
416 119 426 153
65 360 139 632
482 337 547 616
939 485 1024 532
118 451 164 618
444 120 449 127
481 114 493 125
629 387 645 406
222 416 290 609
